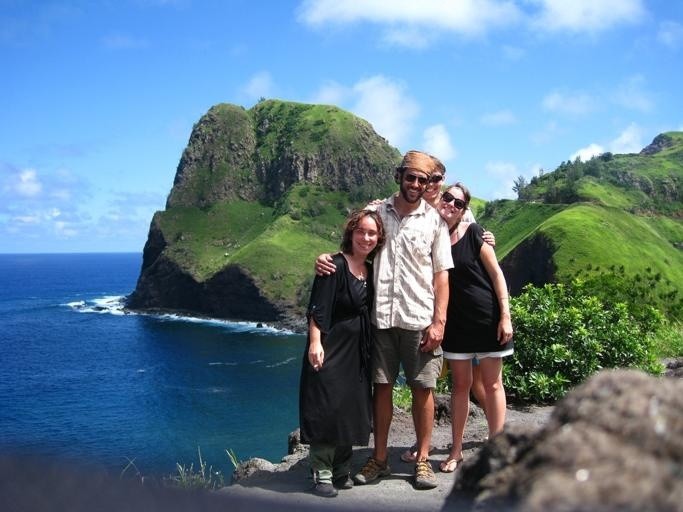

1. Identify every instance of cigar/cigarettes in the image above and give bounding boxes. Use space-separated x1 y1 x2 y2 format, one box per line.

312 363 318 368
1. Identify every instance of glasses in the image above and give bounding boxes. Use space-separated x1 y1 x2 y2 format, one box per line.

401 169 442 186
443 191 466 210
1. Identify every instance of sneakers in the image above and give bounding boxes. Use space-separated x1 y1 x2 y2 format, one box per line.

415 458 438 489
335 475 354 489
401 439 435 463
353 457 391 484
311 475 338 497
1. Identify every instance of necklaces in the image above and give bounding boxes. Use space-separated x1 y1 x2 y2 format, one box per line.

447 222 459 236
359 271 364 276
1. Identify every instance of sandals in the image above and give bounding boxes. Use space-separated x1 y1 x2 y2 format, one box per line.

439 456 463 473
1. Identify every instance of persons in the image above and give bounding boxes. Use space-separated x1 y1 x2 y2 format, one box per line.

367 151 495 465
313 150 454 489
434 183 516 472
298 210 386 497
